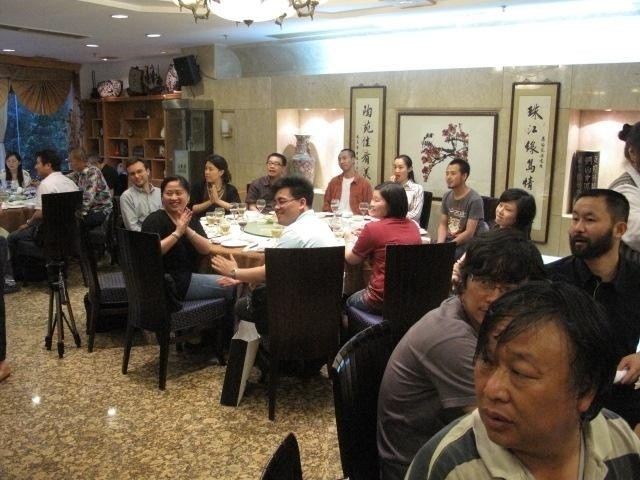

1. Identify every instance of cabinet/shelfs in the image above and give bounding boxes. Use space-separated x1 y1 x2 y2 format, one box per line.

79 94 182 187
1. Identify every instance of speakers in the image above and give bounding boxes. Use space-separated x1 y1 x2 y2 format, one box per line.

172 54 202 86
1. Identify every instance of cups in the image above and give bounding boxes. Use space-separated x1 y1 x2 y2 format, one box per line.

220 221 229 233
331 199 339 217
206 212 216 226
358 202 369 217
30 187 36 199
238 202 247 217
215 208 226 218
271 225 283 238
256 199 266 214
230 203 239 219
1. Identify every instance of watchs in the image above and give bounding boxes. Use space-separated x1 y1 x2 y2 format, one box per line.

171 232 180 240
230 267 240 280
26 220 33 226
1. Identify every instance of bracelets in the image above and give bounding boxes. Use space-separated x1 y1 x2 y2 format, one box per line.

190 230 197 241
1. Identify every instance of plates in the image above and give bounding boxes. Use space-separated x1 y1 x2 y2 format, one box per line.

221 239 248 247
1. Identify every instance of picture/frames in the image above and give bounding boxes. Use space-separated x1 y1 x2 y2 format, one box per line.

396 110 498 202
505 81 561 245
349 86 386 185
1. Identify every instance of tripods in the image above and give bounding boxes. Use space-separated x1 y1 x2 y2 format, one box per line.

44 259 81 358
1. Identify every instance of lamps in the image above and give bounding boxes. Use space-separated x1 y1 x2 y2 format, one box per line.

175 0 320 31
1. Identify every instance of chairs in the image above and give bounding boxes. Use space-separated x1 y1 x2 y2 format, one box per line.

330 319 392 480
237 245 344 420
115 228 227 392
12 192 84 287
85 190 120 265
258 432 303 479
74 218 128 353
349 242 457 347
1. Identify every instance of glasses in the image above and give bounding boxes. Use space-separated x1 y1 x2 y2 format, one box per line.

267 161 281 166
470 271 520 293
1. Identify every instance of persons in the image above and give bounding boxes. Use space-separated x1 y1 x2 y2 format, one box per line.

375 228 545 480
321 149 373 215
0 235 19 293
245 152 287 215
186 155 242 216
405 282 640 480
119 157 165 232
211 176 341 322
0 151 31 192
64 148 113 233
452 188 537 284
437 159 489 280
343 181 422 331
0 293 11 383
455 258 465 267
141 175 235 301
389 155 424 225
607 121 640 280
89 154 119 197
545 188 640 384
7 149 78 281
